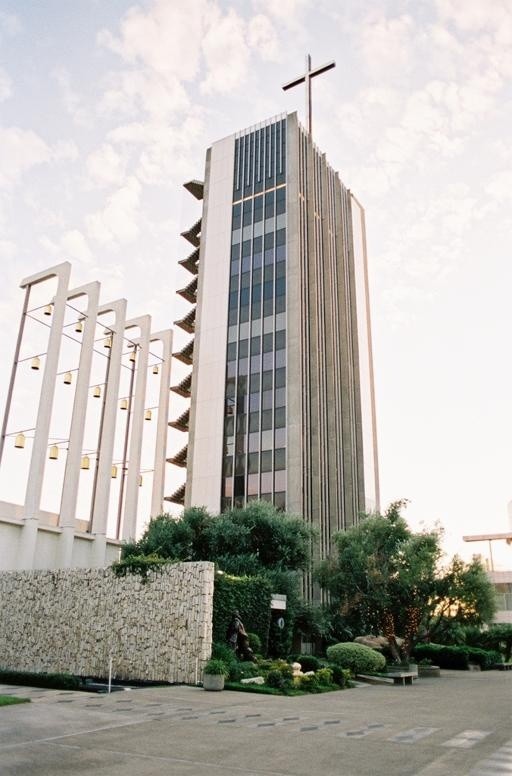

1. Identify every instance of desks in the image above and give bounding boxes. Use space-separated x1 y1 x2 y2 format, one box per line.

494 663 512 670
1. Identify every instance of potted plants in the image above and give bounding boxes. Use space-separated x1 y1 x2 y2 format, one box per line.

202 658 228 691
406 656 419 679
